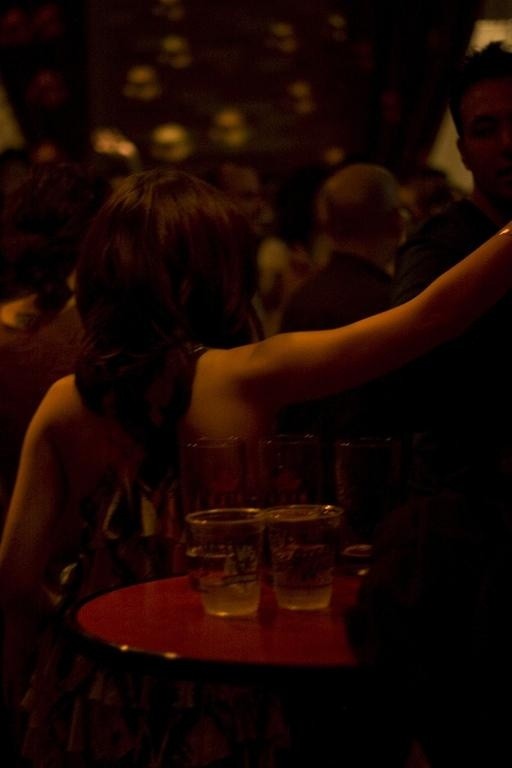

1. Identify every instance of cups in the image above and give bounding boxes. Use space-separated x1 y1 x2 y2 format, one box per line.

187 435 326 590
264 504 345 611
335 436 401 576
186 506 268 619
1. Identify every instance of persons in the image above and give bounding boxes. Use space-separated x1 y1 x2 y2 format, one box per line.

1 169 512 767
0 42 512 488
